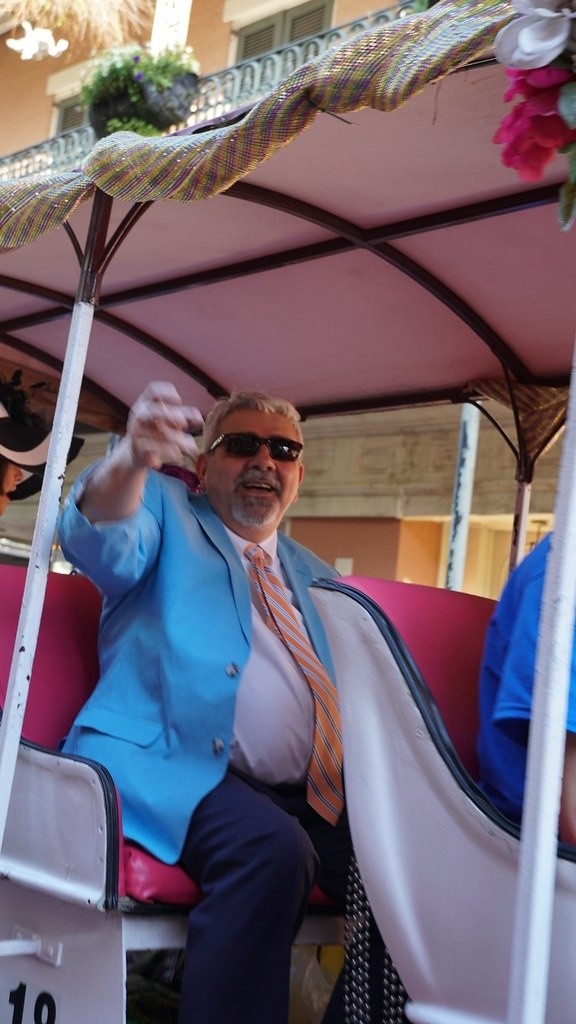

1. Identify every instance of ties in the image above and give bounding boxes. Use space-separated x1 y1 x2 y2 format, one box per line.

242 544 343 826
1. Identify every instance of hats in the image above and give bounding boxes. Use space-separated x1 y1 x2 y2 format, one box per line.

0 370 86 501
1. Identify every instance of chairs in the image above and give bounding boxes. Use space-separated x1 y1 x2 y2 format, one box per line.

0 551 345 1023
308 577 576 1023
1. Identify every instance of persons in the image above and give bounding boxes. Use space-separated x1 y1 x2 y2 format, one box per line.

59 381 350 1023
477 525 575 845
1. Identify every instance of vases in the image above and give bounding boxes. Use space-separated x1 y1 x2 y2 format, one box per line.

89 74 200 136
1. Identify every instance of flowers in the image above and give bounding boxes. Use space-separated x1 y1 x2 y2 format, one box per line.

493 1 576 234
82 45 200 136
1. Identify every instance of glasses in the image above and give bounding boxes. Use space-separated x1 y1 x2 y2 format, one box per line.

208 432 305 461
